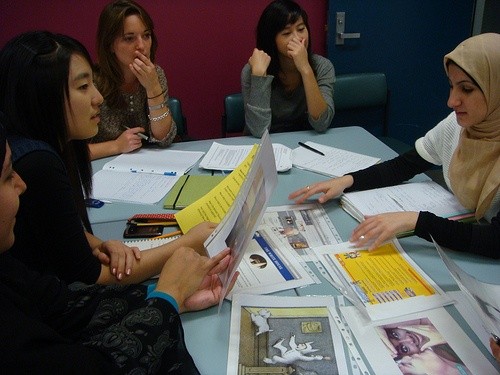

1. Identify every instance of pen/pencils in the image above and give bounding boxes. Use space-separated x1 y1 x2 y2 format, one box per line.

131 170 177 175
298 141 325 156
137 222 178 226
151 229 183 238
123 125 148 140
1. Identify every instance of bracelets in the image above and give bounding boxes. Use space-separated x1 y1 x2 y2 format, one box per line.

145 284 179 312
146 89 163 99
148 101 166 109
148 109 170 121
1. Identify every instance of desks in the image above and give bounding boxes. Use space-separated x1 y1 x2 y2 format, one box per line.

83 126 500 374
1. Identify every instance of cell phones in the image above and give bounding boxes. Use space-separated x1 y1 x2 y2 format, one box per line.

123 225 164 238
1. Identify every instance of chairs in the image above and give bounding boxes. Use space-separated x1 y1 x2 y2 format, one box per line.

222 93 246 138
333 72 388 137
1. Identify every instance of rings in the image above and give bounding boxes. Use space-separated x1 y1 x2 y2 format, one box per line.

359 235 364 240
306 184 310 191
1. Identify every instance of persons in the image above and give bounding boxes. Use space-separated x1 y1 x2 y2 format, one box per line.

89 0 178 161
288 31 500 264
240 0 336 138
0 125 241 375
489 336 500 361
373 317 472 375
0 28 221 290
